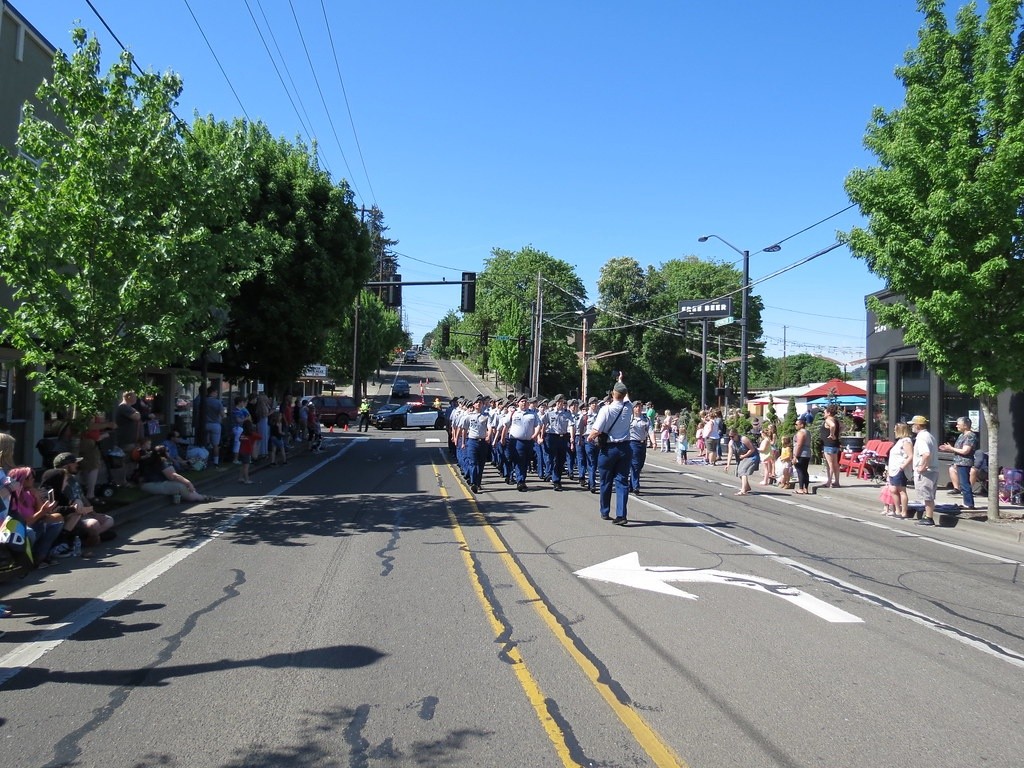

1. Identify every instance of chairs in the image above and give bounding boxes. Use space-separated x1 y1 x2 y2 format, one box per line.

838 439 882 479
845 442 896 480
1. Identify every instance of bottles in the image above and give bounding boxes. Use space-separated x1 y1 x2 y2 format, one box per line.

73 536 81 557
173 488 180 504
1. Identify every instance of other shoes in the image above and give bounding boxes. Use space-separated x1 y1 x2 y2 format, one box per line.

881 509 889 515
38 558 66 569
886 511 896 518
677 452 839 497
203 445 328 486
600 511 611 520
0 605 12 610
458 458 644 496
611 514 629 524
55 545 76 558
0 608 12 617
893 513 908 520
947 488 962 496
958 504 976 513
202 493 225 502
914 519 936 528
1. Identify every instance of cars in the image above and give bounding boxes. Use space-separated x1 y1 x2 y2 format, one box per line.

370 404 401 426
391 380 412 399
305 396 357 428
403 350 419 363
375 404 447 430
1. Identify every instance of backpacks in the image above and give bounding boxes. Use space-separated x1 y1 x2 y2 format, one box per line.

34 420 70 468
718 418 727 439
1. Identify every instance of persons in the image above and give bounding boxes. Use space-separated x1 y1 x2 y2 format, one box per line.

880 420 914 518
446 393 657 495
431 398 443 413
907 415 938 527
356 397 372 433
586 371 630 525
659 407 867 497
0 387 324 583
939 417 988 509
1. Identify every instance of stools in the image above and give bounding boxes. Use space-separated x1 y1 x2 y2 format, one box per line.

973 478 989 497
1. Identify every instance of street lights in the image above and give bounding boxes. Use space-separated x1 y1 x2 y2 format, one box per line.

696 233 783 409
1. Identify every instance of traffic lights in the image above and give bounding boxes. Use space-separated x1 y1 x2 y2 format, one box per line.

518 334 525 351
442 325 449 347
481 329 488 347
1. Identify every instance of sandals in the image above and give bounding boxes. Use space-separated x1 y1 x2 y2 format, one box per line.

88 495 108 507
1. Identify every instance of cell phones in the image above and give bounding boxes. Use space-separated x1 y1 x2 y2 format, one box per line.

48 489 54 505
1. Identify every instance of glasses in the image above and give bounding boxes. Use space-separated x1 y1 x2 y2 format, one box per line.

795 423 802 426
823 412 830 416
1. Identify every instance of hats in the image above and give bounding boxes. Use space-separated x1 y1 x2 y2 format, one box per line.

53 450 84 468
906 415 929 426
613 383 628 395
448 395 652 408
